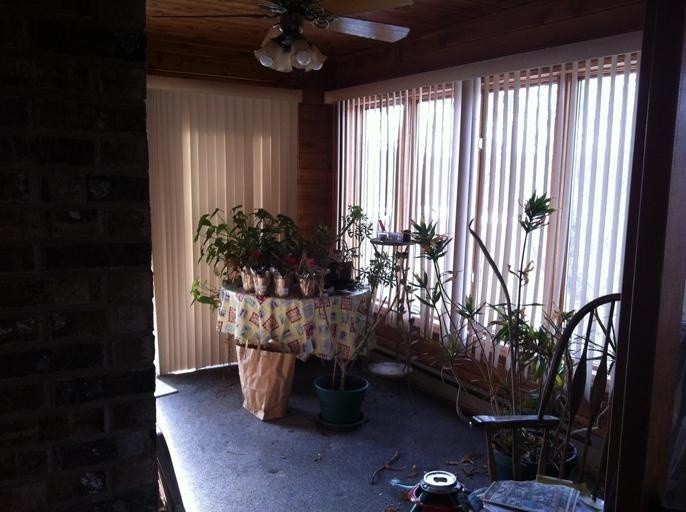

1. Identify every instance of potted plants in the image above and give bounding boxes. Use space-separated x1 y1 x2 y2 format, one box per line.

312 203 404 429
405 188 616 482
187 200 376 298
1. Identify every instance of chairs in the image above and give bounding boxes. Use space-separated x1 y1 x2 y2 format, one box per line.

467 293 617 482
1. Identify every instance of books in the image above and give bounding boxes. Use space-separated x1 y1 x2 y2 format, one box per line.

479 479 581 511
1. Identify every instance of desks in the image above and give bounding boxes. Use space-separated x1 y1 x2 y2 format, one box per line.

215 278 377 366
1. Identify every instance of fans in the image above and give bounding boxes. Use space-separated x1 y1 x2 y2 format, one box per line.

148 0 411 44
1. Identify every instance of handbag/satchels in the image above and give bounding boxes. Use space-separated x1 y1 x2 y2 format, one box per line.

235 334 296 419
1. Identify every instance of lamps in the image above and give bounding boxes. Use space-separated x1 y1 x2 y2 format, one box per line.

253 13 329 73
